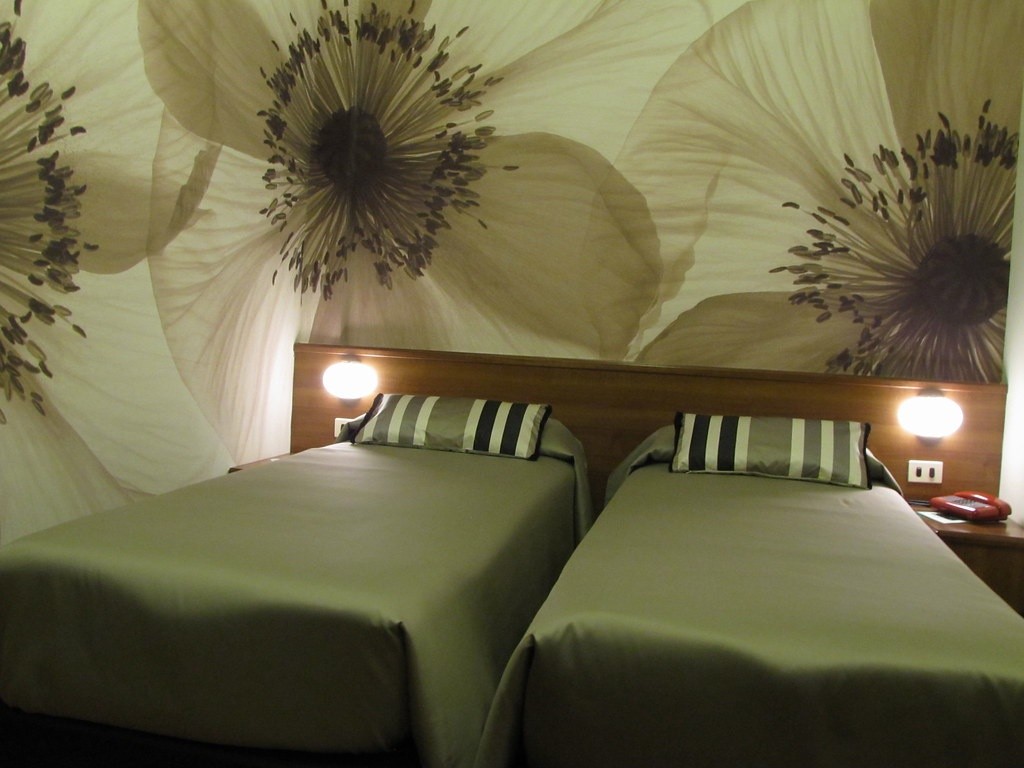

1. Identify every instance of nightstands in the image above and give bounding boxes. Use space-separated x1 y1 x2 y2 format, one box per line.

910 503 1024 617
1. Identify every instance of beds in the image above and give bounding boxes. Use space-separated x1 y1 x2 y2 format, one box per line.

0 343 1024 768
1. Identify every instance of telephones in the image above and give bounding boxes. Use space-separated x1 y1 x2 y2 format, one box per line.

930 491 1012 524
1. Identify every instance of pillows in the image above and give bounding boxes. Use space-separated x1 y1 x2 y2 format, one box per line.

349 393 553 462
669 411 872 489
